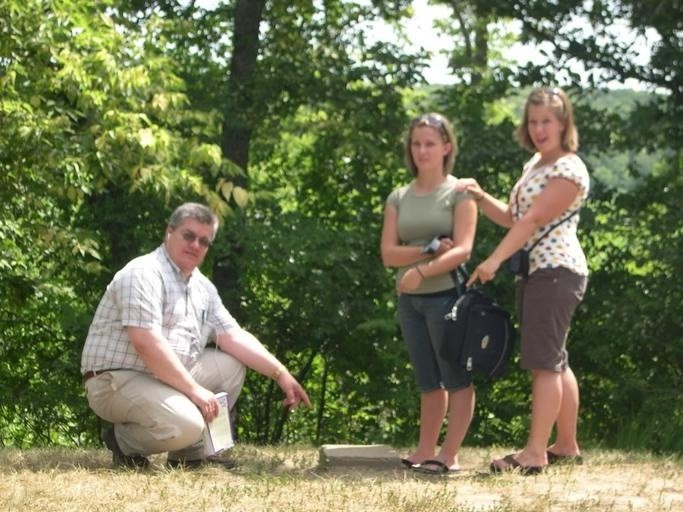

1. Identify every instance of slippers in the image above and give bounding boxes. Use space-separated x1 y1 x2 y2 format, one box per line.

489 450 583 476
401 458 463 474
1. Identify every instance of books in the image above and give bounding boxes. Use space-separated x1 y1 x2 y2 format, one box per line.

201 391 235 460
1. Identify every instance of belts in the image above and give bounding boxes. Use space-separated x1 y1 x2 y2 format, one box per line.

83 369 119 383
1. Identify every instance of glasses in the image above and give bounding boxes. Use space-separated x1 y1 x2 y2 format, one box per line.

181 232 213 247
529 88 566 109
413 112 444 128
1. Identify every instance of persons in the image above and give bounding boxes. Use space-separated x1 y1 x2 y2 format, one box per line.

380 112 479 474
78 202 312 471
455 85 590 475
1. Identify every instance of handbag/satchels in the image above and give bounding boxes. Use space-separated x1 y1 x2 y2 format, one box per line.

508 250 529 278
440 290 515 378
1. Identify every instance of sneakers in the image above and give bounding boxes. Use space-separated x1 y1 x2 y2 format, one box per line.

102 424 149 467
167 458 235 469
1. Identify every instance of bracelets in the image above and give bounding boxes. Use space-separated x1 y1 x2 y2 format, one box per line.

271 365 286 382
415 265 425 280
475 191 483 201
